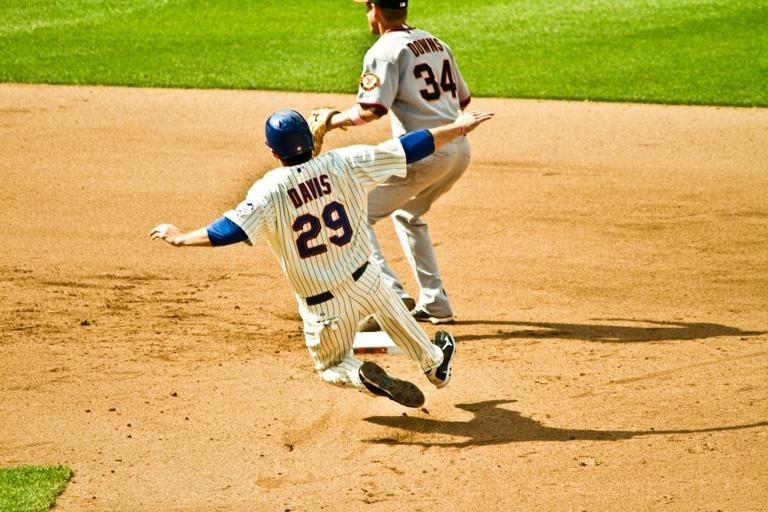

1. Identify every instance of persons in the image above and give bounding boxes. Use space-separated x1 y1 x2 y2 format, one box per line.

147 105 494 409
306 1 473 333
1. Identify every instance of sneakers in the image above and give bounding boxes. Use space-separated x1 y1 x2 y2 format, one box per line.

409 304 453 324
357 361 424 408
358 293 415 332
424 330 455 389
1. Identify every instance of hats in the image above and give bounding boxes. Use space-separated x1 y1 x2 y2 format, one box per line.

353 0 407 9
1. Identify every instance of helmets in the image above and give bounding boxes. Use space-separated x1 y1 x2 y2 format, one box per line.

264 108 313 159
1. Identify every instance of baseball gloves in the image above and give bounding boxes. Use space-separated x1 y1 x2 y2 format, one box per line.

307 104 347 156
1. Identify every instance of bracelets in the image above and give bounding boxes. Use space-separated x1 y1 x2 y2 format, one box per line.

452 120 467 138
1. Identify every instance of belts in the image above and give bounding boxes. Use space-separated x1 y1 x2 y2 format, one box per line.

305 261 367 305
